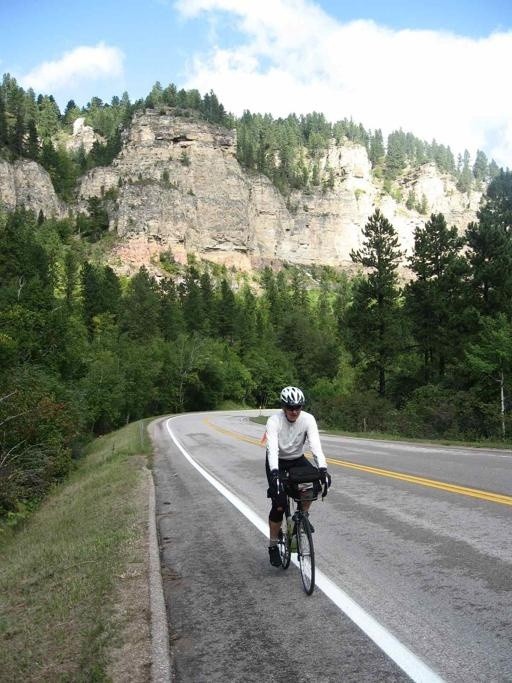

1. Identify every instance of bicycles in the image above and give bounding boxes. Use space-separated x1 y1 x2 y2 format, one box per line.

277 469 330 597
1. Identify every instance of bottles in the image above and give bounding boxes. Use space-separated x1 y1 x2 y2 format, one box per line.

287 514 299 553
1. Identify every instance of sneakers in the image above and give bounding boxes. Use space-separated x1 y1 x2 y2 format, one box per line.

303 512 314 532
269 545 282 566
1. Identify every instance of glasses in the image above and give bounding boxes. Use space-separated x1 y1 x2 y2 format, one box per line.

285 404 302 411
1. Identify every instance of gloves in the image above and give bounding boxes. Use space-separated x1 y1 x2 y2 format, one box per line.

269 470 286 496
319 467 332 488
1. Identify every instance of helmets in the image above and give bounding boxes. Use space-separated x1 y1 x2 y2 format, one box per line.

280 386 306 407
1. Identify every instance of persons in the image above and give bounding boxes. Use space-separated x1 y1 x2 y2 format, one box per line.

264 386 332 567
